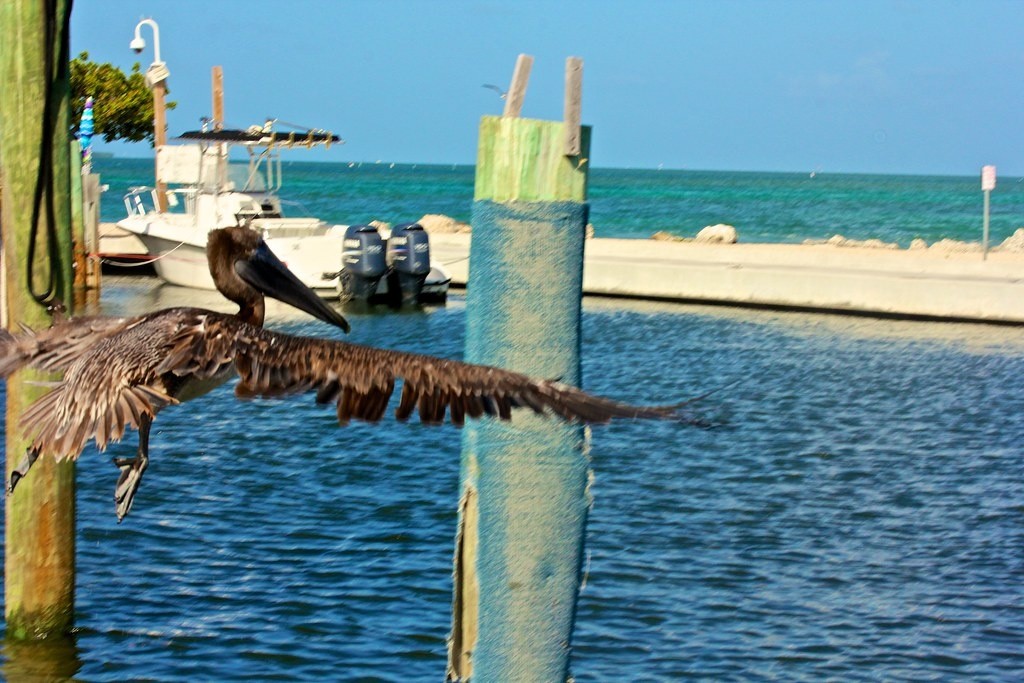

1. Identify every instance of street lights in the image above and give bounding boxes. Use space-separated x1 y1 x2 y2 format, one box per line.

130 18 168 214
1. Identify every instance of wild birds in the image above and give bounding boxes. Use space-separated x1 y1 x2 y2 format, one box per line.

1 222 735 525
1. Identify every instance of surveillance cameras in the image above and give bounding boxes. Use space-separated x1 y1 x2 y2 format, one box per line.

130 37 145 54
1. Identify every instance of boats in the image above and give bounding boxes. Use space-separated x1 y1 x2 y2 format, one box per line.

117 119 451 314
